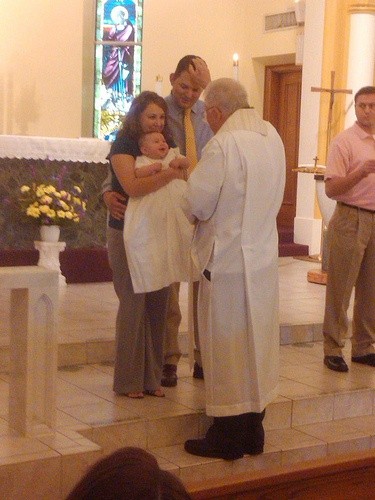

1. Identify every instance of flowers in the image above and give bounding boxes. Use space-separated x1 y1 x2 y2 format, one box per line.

19 181 89 229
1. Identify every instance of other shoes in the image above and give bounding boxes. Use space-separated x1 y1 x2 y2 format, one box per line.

192 362 203 377
117 393 144 399
161 364 178 387
184 437 263 461
144 388 165 397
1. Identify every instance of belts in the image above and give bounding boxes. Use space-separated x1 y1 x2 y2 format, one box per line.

337 202 375 214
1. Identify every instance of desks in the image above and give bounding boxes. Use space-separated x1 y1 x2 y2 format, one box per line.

0 135 111 247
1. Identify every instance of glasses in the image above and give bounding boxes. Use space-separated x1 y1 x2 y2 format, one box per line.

201 106 221 124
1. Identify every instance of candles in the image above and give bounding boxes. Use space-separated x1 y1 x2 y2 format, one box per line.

232 53 239 81
156 75 163 98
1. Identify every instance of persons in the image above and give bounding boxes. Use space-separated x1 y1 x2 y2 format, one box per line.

101 56 215 389
103 6 134 101
322 86 375 372
180 78 286 461
64 446 192 500
105 91 192 398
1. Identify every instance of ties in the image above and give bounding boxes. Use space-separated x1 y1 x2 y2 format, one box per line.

184 109 198 175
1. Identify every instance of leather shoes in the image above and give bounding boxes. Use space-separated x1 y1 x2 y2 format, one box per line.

324 356 349 372
351 353 375 367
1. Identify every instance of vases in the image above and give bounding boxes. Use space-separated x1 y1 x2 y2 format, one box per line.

39 226 60 242
